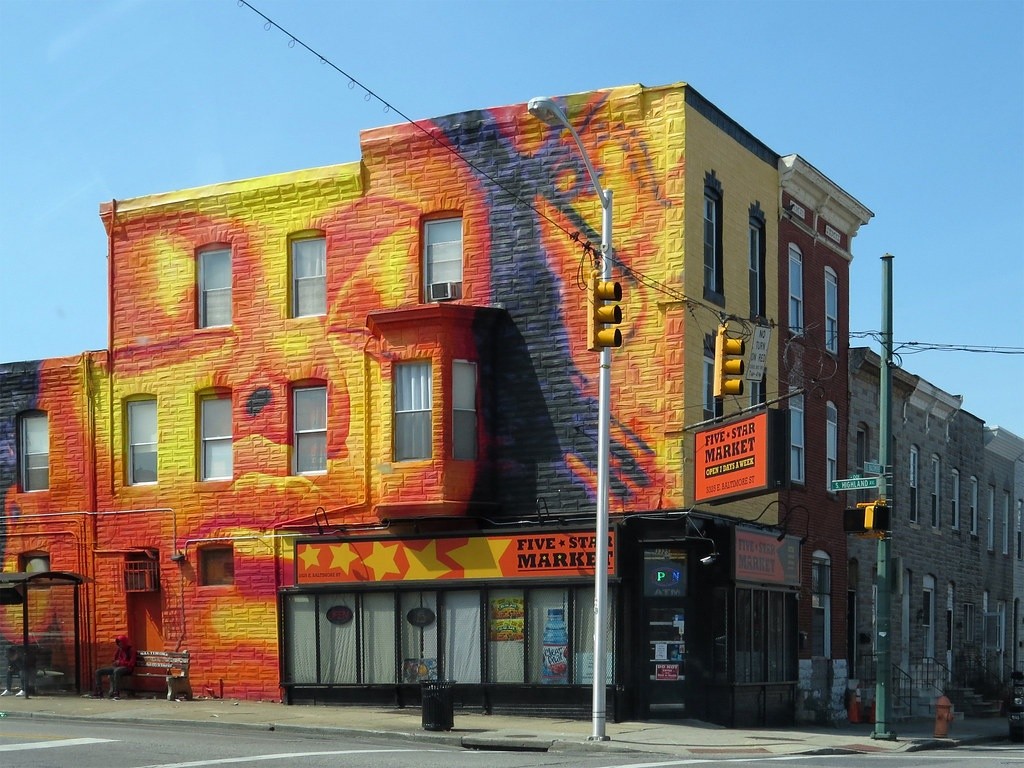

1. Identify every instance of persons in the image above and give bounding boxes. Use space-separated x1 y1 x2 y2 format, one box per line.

1 645 36 697
94 636 136 700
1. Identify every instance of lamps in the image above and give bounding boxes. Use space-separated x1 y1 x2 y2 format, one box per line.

536 497 551 527
775 505 810 545
314 507 331 535
740 500 789 542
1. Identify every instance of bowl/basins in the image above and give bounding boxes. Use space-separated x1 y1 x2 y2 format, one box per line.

170 669 181 676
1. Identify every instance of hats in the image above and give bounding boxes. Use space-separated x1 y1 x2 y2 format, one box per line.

4 647 16 658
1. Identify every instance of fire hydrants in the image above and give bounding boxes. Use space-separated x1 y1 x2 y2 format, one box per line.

935 694 952 737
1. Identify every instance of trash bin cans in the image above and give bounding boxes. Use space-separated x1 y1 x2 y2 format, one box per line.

419 679 458 731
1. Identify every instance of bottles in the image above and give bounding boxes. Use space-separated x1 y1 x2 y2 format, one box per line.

541 608 571 685
180 667 185 676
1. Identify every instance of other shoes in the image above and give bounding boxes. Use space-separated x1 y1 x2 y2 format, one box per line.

1 689 11 696
112 691 121 697
93 693 103 699
15 689 26 697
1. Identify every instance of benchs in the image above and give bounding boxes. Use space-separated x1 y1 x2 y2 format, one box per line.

0 670 65 696
108 649 190 701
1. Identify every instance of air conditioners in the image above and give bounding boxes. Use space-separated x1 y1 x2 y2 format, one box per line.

431 280 457 300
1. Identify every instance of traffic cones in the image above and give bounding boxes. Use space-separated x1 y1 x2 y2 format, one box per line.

869 694 878 723
848 691 863 724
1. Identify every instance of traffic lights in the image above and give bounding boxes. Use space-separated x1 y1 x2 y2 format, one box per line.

871 505 890 530
842 508 866 533
588 277 626 351
715 330 744 400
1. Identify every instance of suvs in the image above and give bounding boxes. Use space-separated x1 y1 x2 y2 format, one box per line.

1008 672 1024 741
